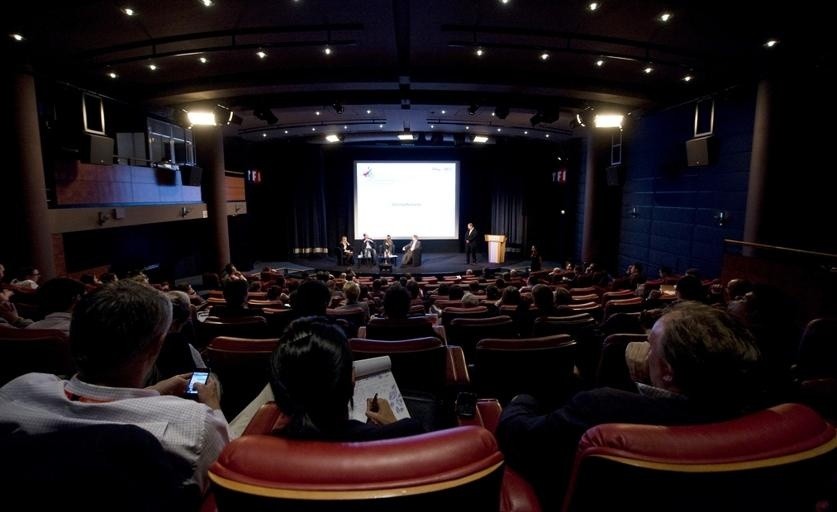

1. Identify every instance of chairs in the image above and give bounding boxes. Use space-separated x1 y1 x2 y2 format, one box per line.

336 247 354 264
1 273 836 512
379 244 395 254
404 246 423 266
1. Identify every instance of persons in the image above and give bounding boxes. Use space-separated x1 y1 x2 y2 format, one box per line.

1 221 837 491
360 233 377 266
341 235 355 267
531 244 542 268
381 235 393 264
399 234 423 268
465 222 479 265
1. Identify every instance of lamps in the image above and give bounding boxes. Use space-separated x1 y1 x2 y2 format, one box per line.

468 103 480 115
253 108 279 127
531 105 559 126
333 103 344 114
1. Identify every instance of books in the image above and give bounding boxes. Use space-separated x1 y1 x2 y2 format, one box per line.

348 354 411 423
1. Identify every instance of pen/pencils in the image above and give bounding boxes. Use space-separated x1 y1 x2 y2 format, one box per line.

367 392 378 425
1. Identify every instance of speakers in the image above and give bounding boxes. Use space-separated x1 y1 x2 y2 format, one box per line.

79 133 114 166
687 136 720 167
607 166 627 186
179 166 203 186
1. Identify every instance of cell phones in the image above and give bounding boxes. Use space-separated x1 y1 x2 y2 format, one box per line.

186 367 210 395
